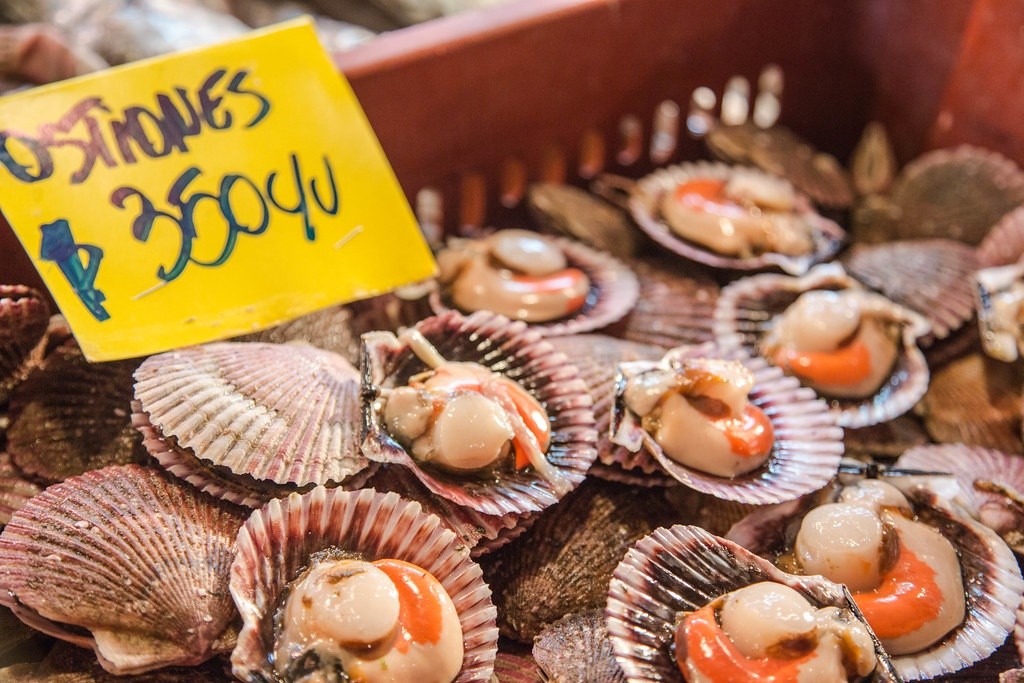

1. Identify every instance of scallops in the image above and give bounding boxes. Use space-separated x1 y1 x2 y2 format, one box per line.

0 117 1023 683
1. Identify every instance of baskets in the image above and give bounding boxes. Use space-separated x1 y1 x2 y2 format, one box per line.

0 0 1024 683
